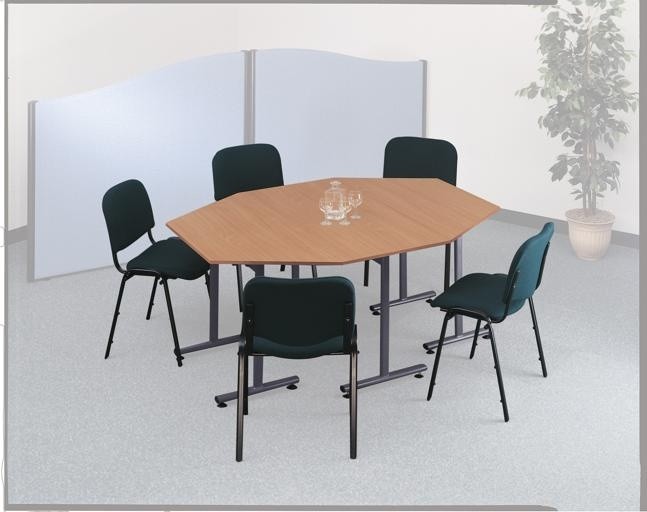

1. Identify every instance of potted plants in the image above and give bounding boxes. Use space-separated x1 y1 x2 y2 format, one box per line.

516 0 643 265
